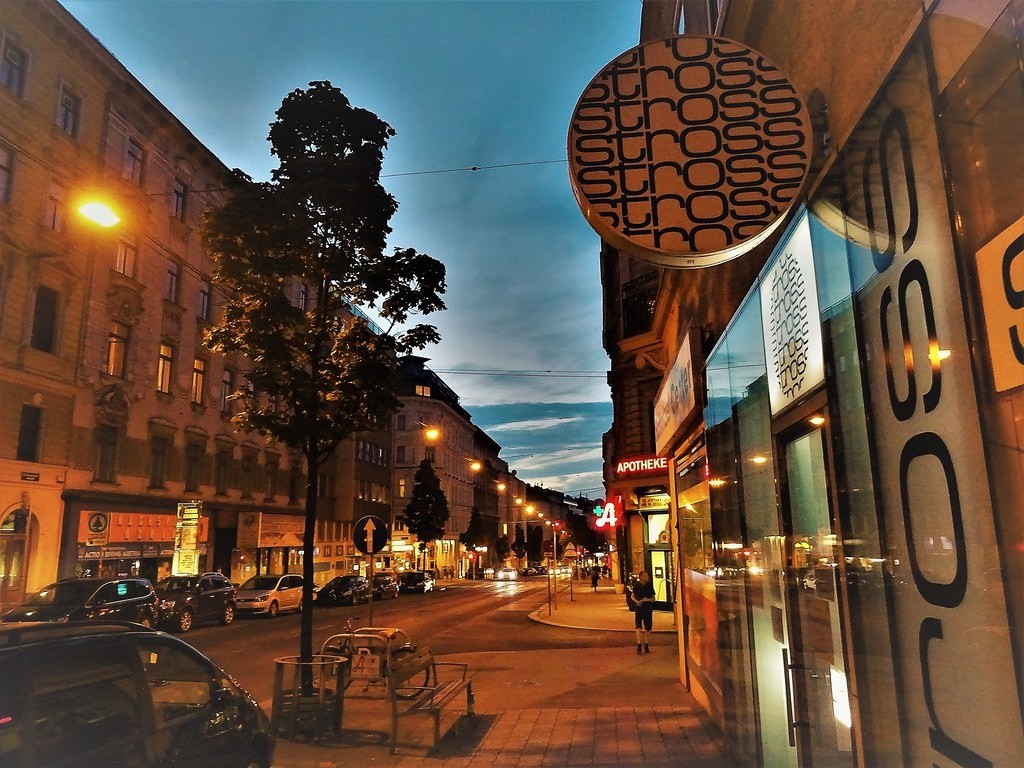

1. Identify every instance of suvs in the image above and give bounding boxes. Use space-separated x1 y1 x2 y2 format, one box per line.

151 571 239 633
0 623 277 768
0 577 162 633
232 571 305 618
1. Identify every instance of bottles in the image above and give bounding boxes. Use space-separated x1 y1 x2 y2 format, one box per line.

639 598 646 604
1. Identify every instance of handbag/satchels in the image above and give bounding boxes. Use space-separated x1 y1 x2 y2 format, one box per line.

591 584 593 588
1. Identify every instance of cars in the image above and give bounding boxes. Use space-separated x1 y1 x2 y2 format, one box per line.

803 566 837 592
402 571 435 595
520 565 547 576
318 575 371 604
373 572 400 600
826 562 868 588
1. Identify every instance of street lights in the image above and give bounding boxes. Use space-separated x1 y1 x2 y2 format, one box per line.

64 190 125 479
550 522 561 610
386 410 442 557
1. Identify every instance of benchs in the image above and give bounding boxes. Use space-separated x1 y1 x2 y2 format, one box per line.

387 647 476 757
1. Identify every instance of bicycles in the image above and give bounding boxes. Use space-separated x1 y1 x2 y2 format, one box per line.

592 575 601 592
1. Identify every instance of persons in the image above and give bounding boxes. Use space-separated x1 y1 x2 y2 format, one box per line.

630 571 656 654
586 565 608 577
590 567 600 592
443 565 455 583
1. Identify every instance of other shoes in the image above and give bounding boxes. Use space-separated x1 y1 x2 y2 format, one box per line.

645 643 649 653
637 644 642 654
